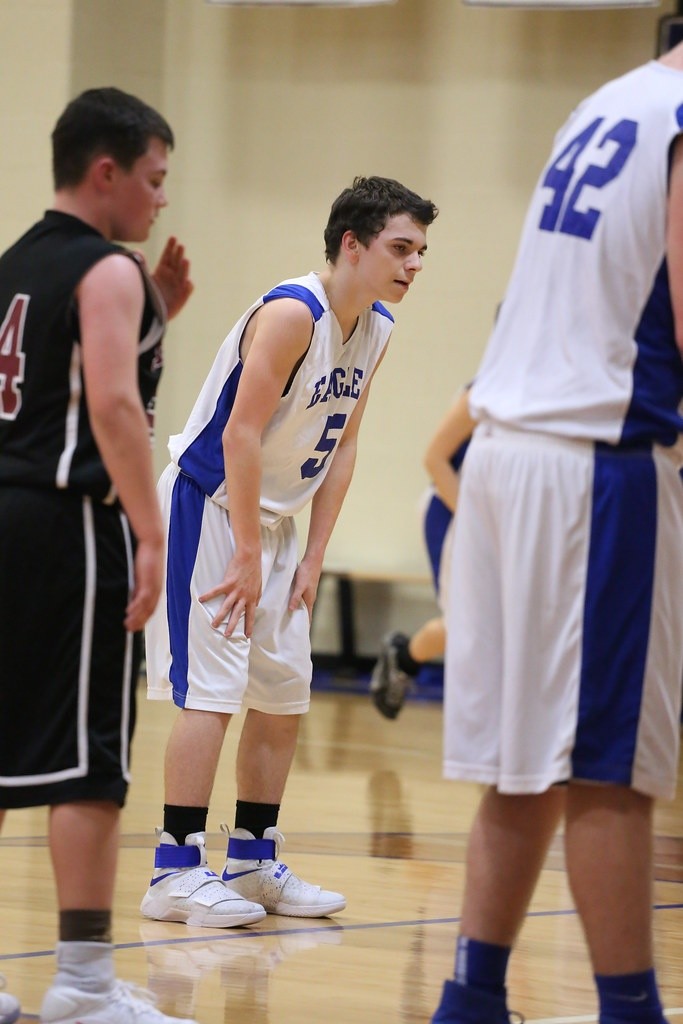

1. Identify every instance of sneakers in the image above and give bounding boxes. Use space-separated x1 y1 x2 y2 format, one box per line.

369 632 415 718
0 993 22 1024
219 823 345 917
38 980 199 1023
139 828 267 928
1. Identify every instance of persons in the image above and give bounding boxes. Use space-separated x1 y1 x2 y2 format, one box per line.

367 374 480 722
4 89 437 1023
426 36 683 1024
